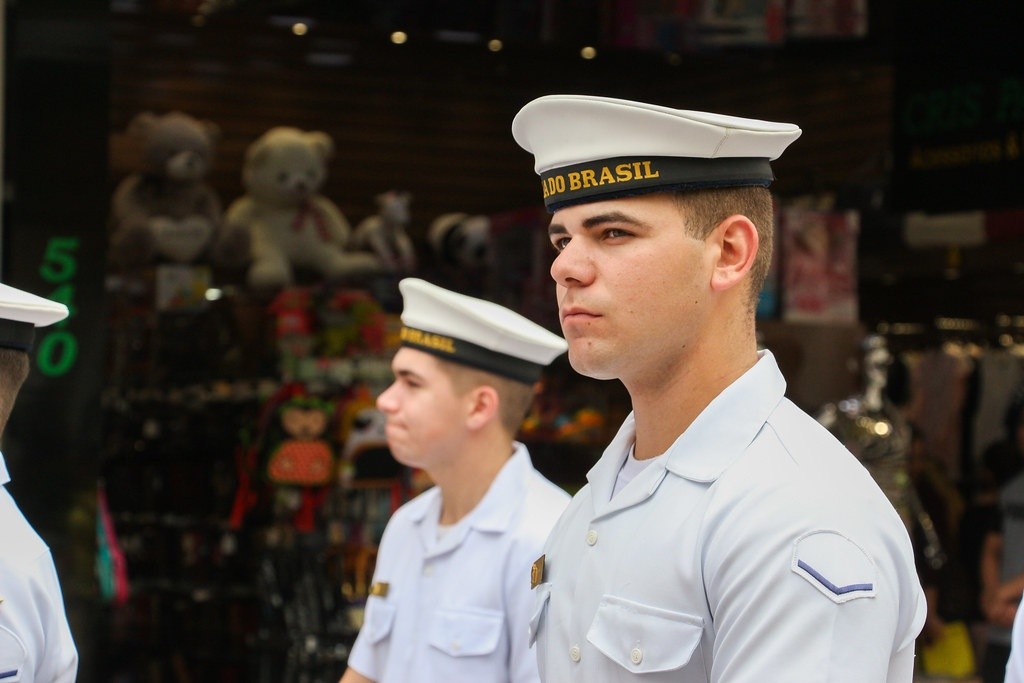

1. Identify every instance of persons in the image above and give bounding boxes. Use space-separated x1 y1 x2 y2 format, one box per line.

904 398 1024 683
510 93 927 683
0 284 88 683
338 279 573 683
817 331 943 571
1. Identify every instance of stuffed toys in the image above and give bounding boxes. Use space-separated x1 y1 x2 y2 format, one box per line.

100 104 502 496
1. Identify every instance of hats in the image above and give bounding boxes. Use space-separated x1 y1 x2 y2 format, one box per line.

399 278 569 385
0 282 69 352
510 95 802 213
